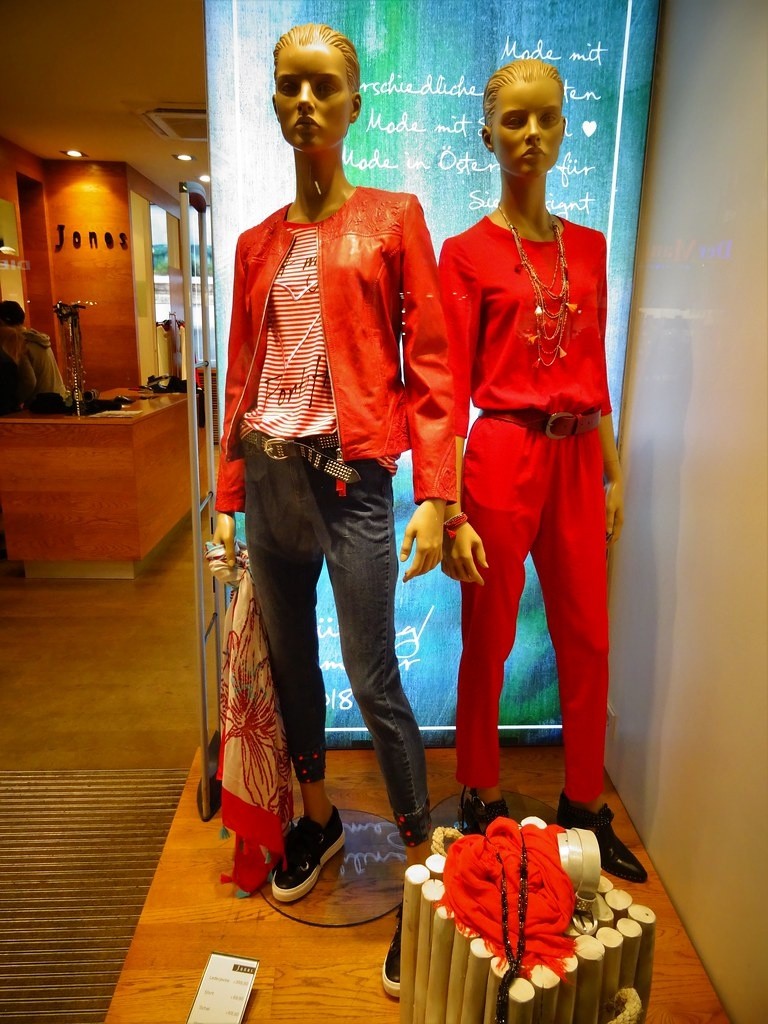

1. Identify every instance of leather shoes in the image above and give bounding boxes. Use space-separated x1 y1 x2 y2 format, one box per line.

464 788 508 834
556 790 649 883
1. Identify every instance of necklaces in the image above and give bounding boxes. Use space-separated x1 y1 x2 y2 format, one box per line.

498 202 577 369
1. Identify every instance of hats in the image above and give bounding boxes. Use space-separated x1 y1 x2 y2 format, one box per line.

0 301 25 326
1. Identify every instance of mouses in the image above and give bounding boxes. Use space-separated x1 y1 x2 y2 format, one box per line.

114 395 132 404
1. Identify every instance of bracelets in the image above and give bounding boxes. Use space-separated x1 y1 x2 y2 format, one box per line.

443 511 468 540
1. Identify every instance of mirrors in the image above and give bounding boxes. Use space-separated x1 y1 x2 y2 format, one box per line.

150 202 176 377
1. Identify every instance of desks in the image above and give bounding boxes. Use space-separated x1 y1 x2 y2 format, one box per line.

0 387 211 580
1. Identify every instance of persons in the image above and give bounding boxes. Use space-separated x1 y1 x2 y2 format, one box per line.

205 23 457 998
439 59 648 884
0 301 67 418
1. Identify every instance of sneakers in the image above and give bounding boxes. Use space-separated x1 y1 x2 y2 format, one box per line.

271 805 345 903
382 884 404 997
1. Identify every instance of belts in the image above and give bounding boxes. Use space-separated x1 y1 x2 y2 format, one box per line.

242 431 362 484
478 408 601 440
